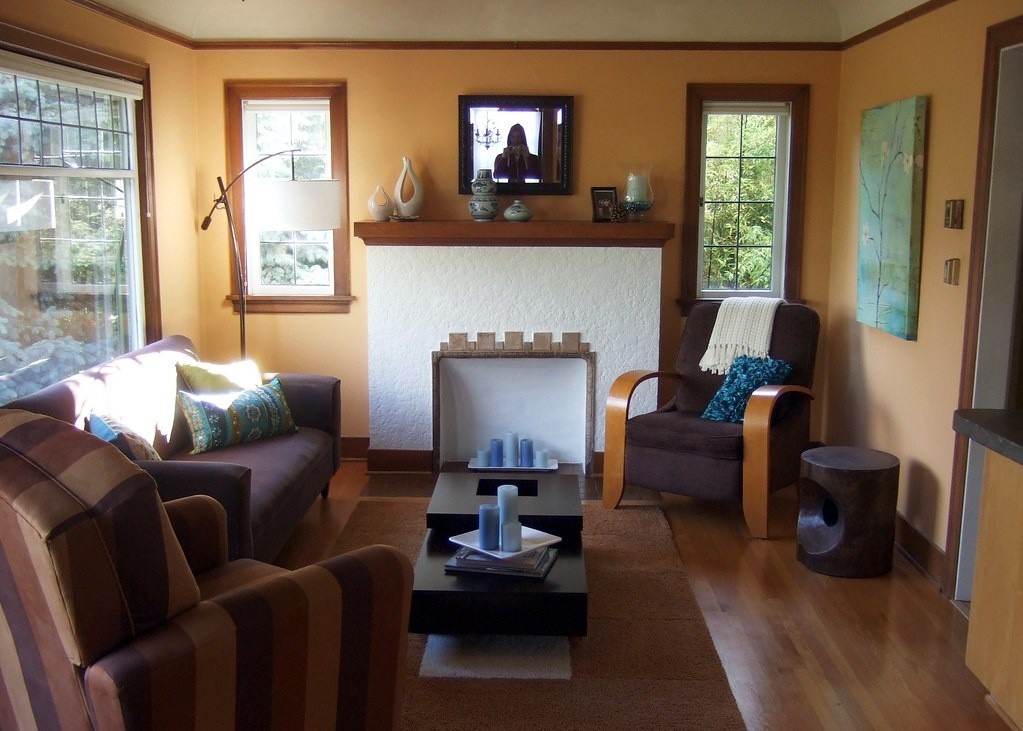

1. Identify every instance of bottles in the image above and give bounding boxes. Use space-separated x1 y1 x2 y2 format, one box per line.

468 169 500 222
503 200 532 221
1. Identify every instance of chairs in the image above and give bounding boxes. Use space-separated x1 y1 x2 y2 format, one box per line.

601 301 821 541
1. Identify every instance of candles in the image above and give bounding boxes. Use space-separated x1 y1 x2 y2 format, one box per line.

500 521 522 552
535 448 549 467
626 175 648 202
520 439 534 467
476 448 490 467
497 485 519 546
479 504 500 551
490 438 504 467
505 430 518 467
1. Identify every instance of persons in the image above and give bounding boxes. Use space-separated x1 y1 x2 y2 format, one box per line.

494 124 540 179
598 198 614 217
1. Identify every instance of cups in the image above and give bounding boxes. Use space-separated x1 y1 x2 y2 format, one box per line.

618 161 654 222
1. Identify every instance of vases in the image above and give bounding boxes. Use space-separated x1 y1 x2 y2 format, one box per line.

368 185 395 222
393 156 425 217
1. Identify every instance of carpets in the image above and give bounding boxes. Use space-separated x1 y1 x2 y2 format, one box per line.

333 500 748 731
417 636 573 680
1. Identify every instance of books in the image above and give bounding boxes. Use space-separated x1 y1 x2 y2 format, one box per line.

444 544 558 578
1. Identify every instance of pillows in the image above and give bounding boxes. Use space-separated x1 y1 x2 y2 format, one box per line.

89 407 161 461
176 358 263 394
176 377 300 455
701 354 793 424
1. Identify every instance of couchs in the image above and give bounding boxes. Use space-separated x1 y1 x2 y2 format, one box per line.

0 407 415 731
0 334 342 565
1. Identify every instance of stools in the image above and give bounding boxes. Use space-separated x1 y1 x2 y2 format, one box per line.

797 446 901 580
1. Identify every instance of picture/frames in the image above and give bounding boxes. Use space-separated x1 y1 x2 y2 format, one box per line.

590 186 619 223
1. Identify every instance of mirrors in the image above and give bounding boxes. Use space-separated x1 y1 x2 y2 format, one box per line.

458 94 575 197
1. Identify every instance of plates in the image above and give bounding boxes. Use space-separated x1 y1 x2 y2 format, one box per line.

468 457 559 470
449 526 563 559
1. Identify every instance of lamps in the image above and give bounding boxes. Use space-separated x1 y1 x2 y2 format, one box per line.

0 162 128 337
475 109 504 150
200 148 342 361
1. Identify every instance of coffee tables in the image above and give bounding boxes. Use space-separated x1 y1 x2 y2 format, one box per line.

407 472 589 640
